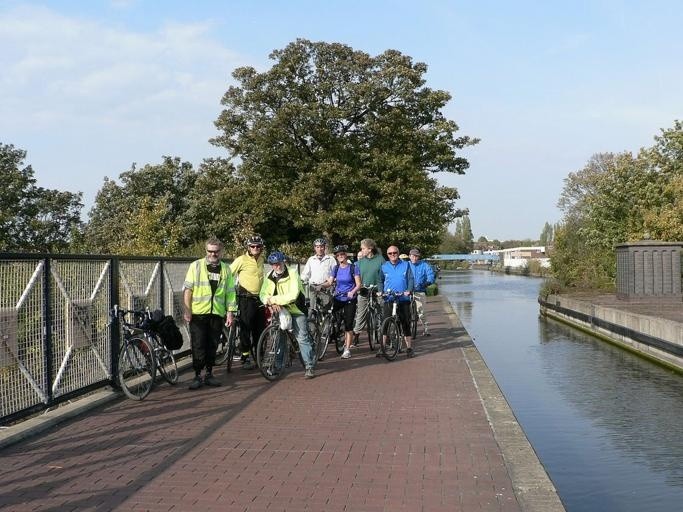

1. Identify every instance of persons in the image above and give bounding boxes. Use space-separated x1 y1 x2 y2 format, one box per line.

228 235 265 370
300 238 337 344
355 246 364 261
259 251 317 379
319 246 362 359
376 245 414 359
183 239 238 390
349 238 386 349
408 249 435 338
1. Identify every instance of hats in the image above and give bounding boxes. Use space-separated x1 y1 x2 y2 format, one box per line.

408 249 421 256
332 245 347 253
312 238 326 246
267 252 285 264
245 236 263 245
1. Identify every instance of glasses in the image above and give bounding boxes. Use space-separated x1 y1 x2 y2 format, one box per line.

387 251 399 255
205 249 220 254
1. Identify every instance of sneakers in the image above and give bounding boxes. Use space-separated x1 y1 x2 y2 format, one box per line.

375 346 385 358
187 378 201 390
304 366 314 380
422 328 431 338
405 348 415 358
349 338 361 349
240 357 254 372
204 375 222 387
340 349 351 360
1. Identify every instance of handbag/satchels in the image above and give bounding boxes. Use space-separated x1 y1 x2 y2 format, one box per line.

156 314 183 350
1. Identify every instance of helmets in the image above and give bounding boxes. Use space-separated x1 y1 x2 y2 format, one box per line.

399 253 409 259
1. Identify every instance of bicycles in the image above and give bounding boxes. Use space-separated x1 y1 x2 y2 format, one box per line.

108 304 178 400
211 284 423 381
